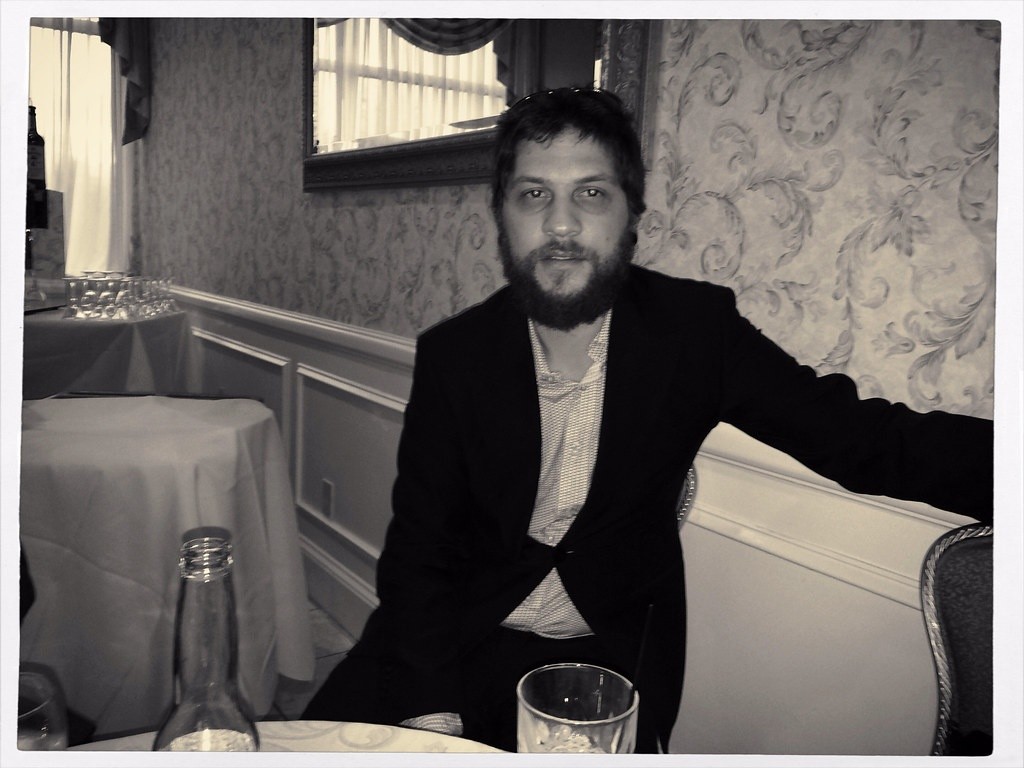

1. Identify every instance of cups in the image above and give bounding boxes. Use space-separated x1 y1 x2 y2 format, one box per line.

516 662 640 754
18 662 67 750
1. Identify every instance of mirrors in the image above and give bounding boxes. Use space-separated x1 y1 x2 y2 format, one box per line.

302 18 663 195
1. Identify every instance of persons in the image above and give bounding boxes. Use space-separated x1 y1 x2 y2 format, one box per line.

299 85 993 753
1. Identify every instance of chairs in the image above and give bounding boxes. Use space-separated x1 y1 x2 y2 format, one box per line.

919 522 994 756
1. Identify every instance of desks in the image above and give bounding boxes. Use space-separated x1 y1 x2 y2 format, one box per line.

23 304 188 400
20 395 315 741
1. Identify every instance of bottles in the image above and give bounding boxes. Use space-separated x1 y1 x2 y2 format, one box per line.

26 105 47 227
148 527 260 752
